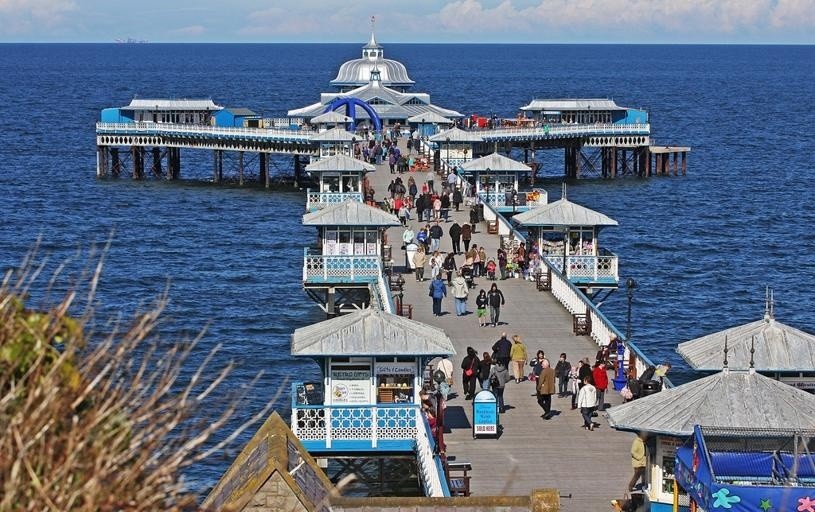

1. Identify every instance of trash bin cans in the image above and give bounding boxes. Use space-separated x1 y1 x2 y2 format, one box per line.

643 380 659 397
475 204 483 220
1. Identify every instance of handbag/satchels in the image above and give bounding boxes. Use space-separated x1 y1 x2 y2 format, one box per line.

489 374 500 387
447 378 453 386
429 287 434 297
465 370 473 376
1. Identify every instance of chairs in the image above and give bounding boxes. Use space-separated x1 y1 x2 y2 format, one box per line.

601 341 618 370
487 221 499 234
536 268 551 291
572 313 592 336
614 354 636 379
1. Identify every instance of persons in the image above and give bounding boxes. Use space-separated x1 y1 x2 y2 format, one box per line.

353 111 527 328
651 362 671 386
639 365 655 382
437 357 454 388
461 332 617 431
418 390 437 438
627 434 646 492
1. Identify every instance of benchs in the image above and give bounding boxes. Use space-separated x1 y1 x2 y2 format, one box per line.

445 464 471 497
396 296 412 320
437 165 445 175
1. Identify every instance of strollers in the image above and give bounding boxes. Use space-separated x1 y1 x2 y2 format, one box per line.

484 256 497 281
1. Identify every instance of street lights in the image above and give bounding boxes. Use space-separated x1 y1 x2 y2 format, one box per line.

511 187 516 216
562 229 568 277
344 117 356 158
154 105 264 129
369 188 375 206
434 366 445 456
489 105 591 125
379 226 388 274
626 277 634 341
363 168 368 200
396 273 406 317
420 117 489 203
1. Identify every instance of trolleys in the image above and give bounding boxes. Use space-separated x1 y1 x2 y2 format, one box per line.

472 390 504 441
